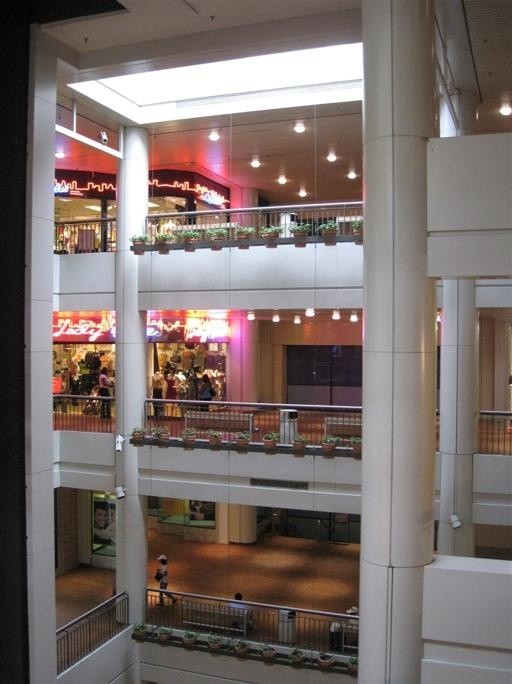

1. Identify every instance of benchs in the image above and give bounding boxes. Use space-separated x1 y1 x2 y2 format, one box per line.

336 213 366 240
323 415 363 445
180 599 253 640
183 408 262 443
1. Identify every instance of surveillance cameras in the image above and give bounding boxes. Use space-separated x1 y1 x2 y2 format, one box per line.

115 435 123 452
100 130 108 144
450 513 462 530
115 487 125 500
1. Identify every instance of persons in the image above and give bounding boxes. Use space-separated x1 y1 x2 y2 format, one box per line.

94 502 111 545
54 351 216 422
328 606 359 652
228 591 256 636
154 553 178 607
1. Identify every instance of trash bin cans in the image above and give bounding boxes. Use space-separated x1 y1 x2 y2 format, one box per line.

279 610 297 644
279 409 298 444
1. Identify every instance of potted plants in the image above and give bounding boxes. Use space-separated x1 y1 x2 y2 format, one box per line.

132 620 359 679
130 219 368 255
131 425 364 460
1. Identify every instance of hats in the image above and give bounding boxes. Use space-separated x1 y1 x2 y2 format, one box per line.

345 607 358 614
157 555 167 560
